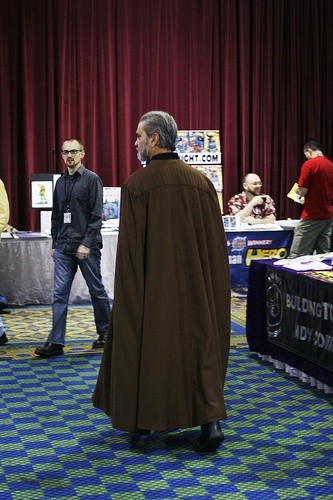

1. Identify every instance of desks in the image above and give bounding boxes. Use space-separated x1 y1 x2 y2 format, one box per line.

225 226 294 298
245 258 333 395
0 232 119 306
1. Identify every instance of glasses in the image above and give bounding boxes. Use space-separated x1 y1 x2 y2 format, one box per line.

61 149 83 156
245 181 263 187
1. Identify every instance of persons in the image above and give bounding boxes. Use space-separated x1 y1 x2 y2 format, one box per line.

90 110 231 453
228 172 276 225
289 139 333 258
0 177 10 347
35 139 112 356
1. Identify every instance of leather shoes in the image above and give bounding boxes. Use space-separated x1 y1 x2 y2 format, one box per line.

36 342 65 356
93 333 107 348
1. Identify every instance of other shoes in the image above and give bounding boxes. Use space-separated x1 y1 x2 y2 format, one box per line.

131 431 173 451
194 421 225 454
231 288 247 298
0 333 8 344
